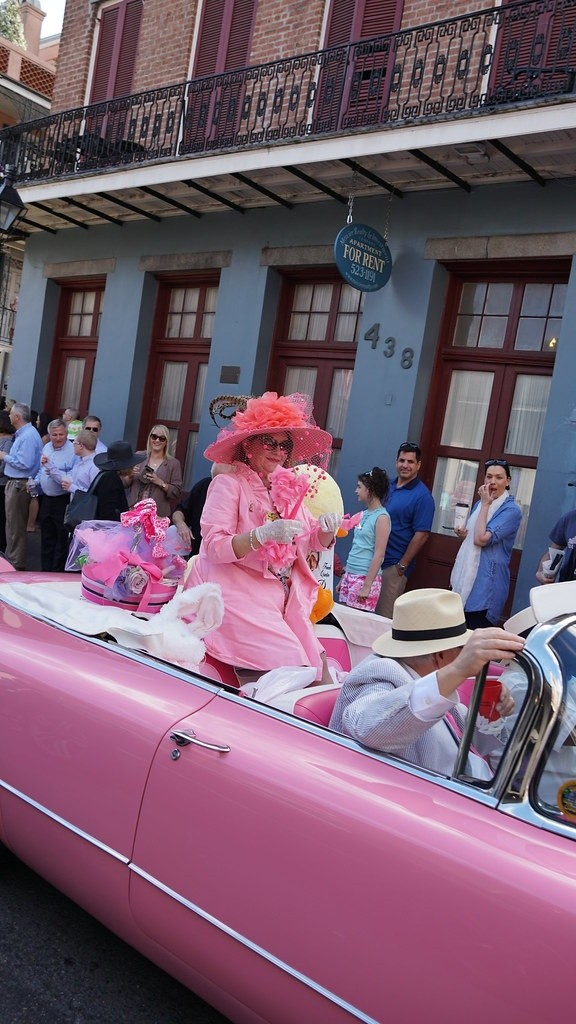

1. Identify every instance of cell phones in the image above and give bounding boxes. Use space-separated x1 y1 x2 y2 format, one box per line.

139 465 154 484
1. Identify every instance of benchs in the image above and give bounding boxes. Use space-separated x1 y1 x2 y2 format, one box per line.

282 671 505 737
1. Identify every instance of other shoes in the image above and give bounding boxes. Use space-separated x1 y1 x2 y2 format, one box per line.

27 527 36 533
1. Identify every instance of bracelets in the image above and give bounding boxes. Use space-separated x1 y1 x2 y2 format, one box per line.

250 529 258 551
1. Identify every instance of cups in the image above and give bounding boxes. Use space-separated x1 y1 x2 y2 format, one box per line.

478 680 502 725
454 503 470 529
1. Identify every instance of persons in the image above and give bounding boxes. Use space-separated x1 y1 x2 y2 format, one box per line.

181 391 344 687
496 582 576 809
0 395 231 573
330 588 526 780
535 481 576 583
334 443 434 619
448 459 522 629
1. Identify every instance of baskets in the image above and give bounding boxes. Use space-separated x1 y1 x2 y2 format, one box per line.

73 498 191 614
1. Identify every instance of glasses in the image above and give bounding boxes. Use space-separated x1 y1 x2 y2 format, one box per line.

150 433 166 442
364 470 373 481
84 427 99 433
259 436 294 455
401 442 417 448
484 458 507 466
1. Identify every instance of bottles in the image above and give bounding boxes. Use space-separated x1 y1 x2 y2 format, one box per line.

28 477 38 498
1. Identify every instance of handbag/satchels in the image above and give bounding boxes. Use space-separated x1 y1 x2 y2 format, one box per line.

64 473 104 532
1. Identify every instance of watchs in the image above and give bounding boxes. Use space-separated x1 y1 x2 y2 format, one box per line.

397 563 407 572
161 480 166 488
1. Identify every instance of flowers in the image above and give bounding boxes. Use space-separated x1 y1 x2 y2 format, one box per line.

112 565 148 596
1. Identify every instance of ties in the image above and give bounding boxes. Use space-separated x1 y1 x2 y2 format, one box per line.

445 706 480 757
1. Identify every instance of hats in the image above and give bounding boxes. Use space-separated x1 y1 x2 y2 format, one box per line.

202 394 333 465
568 480 576 487
93 440 147 471
371 587 475 659
66 420 85 439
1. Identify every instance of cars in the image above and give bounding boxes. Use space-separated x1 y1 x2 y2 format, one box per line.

0 552 576 1024
